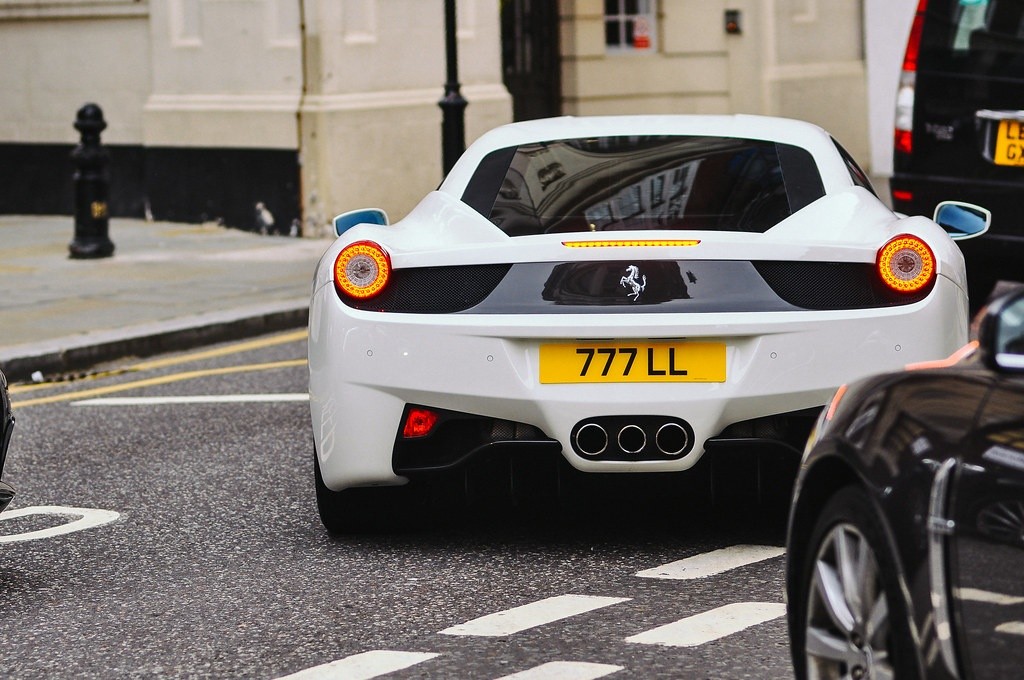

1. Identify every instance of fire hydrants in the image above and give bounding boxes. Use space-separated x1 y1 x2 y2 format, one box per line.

71 105 116 258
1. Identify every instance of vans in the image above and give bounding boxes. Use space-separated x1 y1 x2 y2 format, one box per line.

890 0 1024 311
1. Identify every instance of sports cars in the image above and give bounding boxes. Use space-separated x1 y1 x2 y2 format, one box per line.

786 286 1024 680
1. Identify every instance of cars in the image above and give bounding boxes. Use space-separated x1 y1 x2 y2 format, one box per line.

305 113 992 536
